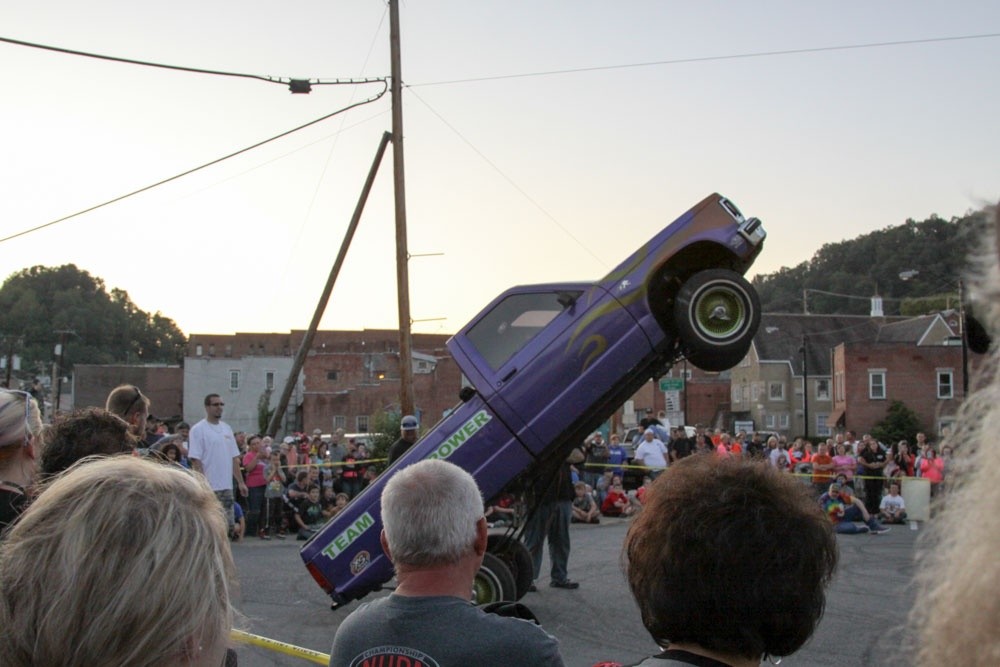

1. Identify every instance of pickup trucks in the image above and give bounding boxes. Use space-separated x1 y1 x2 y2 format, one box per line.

296 192 768 611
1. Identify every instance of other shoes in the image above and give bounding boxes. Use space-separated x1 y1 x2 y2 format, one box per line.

571 517 580 523
591 518 600 524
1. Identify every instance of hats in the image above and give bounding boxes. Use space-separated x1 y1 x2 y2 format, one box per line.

645 408 653 414
283 436 294 443
401 415 419 431
676 425 684 430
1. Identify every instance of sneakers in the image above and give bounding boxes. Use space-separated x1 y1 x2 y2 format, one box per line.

551 578 579 588
231 533 241 542
855 526 869 533
274 529 286 540
258 527 270 539
871 523 891 534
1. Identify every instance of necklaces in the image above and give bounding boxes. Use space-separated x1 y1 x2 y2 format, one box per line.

209 422 224 433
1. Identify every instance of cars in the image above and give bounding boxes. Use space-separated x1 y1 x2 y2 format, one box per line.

617 424 698 465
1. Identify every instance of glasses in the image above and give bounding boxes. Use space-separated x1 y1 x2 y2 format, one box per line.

208 403 225 406
0 389 32 447
124 387 142 415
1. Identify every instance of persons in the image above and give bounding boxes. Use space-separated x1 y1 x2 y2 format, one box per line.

388 415 420 464
521 449 580 591
137 414 379 541
605 451 840 667
0 456 233 667
188 394 248 533
329 458 564 667
40 407 137 485
564 408 973 534
106 385 161 461
0 387 43 543
